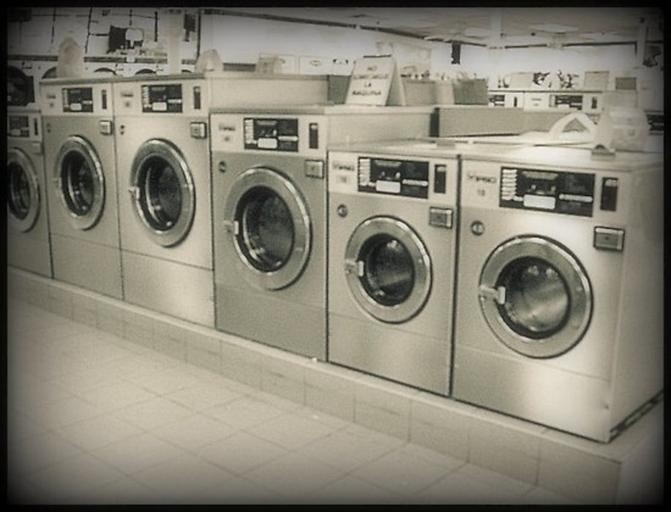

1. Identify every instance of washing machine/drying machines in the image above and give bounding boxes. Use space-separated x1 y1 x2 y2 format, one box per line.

3 71 666 448
